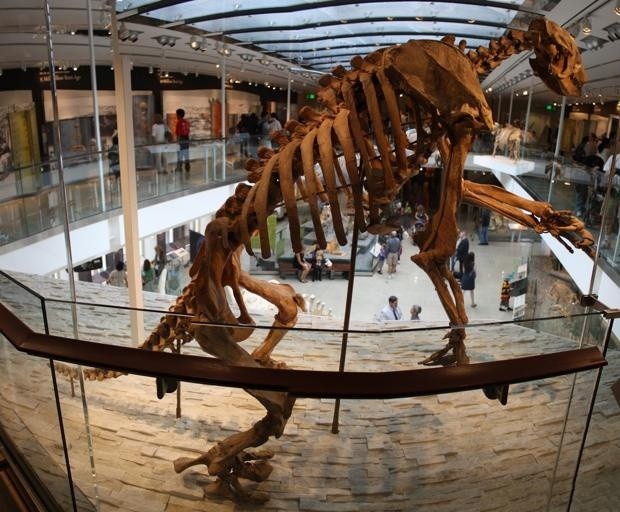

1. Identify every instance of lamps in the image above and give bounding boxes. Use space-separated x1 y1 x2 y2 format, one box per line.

120 30 255 65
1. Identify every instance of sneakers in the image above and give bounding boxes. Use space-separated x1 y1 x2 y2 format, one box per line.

498 305 513 312
471 303 477 309
156 162 192 175
299 275 323 284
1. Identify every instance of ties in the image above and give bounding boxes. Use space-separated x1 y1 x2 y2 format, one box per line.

392 307 399 321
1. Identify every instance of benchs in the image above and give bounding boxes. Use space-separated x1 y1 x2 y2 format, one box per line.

277 258 351 279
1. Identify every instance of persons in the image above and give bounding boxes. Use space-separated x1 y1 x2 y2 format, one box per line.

172 108 191 173
107 135 120 180
142 260 155 291
571 131 620 249
380 295 403 320
236 112 283 158
152 246 164 277
295 200 429 282
478 208 491 245
107 261 128 287
460 251 479 307
152 114 169 175
1 136 12 172
410 304 421 320
453 230 469 276
